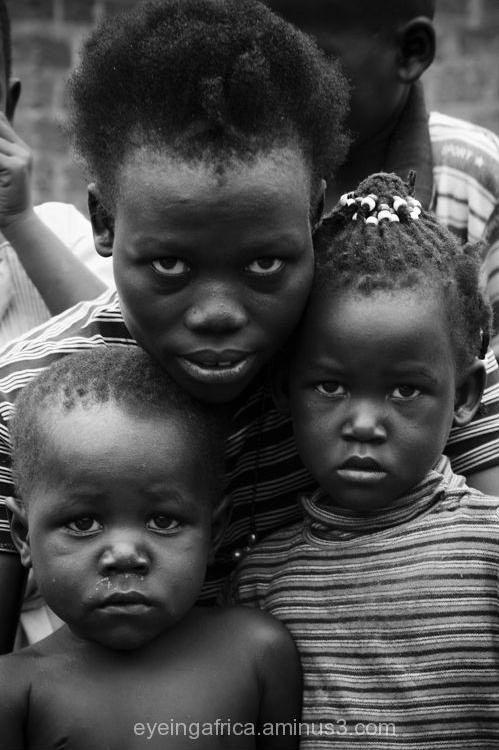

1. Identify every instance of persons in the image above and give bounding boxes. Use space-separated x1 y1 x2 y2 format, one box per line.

0 345 302 749
0 3 135 654
265 0 497 345
224 170 499 747
0 4 498 657
